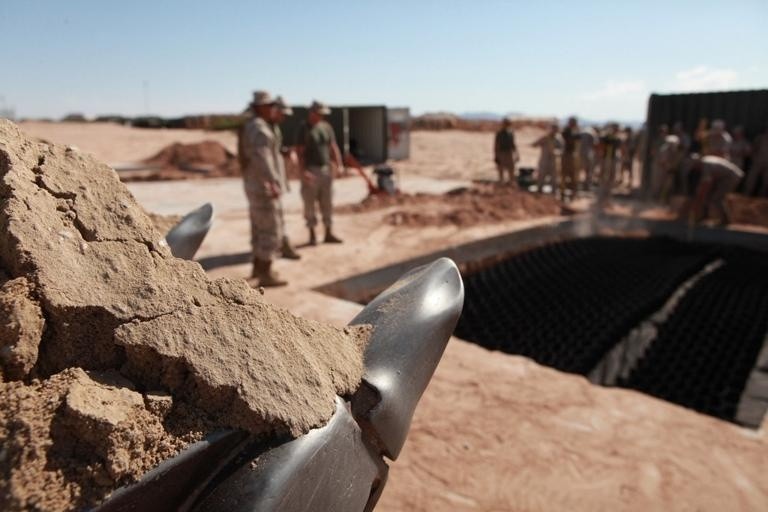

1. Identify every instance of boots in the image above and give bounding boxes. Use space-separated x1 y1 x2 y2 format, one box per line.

250 228 342 288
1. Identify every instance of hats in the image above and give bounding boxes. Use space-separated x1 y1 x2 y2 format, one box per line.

249 90 332 116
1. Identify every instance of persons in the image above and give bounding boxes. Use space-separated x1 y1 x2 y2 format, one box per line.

297 100 349 245
239 91 287 290
492 113 521 187
531 118 767 229
272 99 302 262
